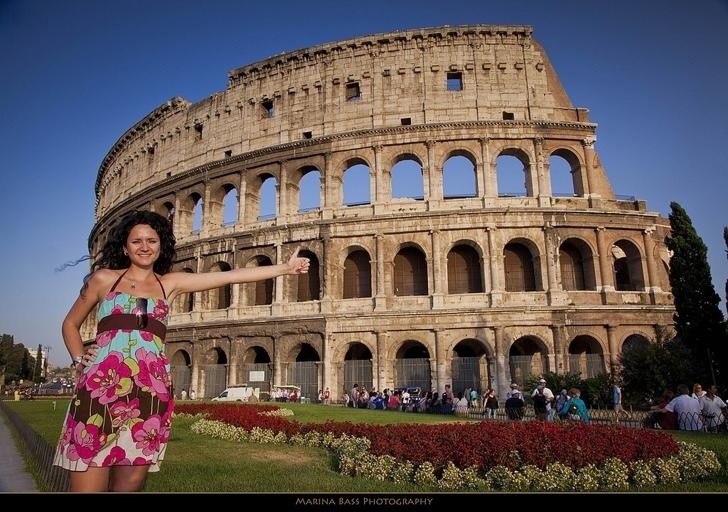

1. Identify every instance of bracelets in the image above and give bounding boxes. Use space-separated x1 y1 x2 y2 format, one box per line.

72 351 87 365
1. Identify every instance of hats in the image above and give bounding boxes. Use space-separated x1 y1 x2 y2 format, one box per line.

511 378 548 395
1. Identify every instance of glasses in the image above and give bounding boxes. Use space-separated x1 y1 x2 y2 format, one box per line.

135 297 148 330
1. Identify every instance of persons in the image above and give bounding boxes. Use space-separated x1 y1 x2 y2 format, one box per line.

641 381 728 432
13 386 26 401
609 381 633 423
189 389 196 400
179 388 188 400
268 377 591 426
49 208 313 491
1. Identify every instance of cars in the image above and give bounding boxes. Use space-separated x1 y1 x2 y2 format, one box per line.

394 385 422 402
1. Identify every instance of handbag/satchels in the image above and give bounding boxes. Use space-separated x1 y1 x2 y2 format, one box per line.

566 403 578 417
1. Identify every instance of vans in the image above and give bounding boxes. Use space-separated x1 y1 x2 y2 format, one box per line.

210 386 254 402
